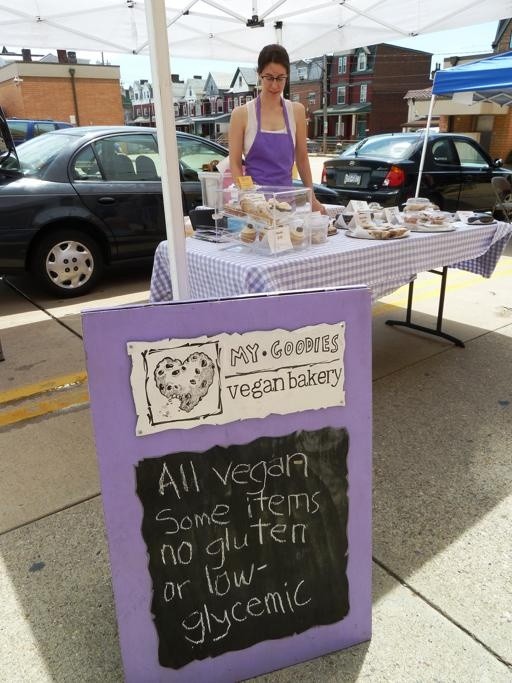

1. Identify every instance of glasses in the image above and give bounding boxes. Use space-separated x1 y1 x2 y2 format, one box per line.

259 73 288 82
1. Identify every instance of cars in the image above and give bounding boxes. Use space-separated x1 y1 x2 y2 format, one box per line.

321 132 512 221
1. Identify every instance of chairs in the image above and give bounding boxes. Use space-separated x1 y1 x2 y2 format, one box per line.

115 153 157 179
434 144 449 163
492 176 510 222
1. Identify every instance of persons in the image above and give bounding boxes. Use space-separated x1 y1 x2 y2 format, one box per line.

227 43 327 219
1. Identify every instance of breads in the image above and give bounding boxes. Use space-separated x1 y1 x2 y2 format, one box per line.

154 352 214 412
235 195 445 244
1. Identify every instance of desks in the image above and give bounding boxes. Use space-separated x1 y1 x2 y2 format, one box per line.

159 213 510 352
